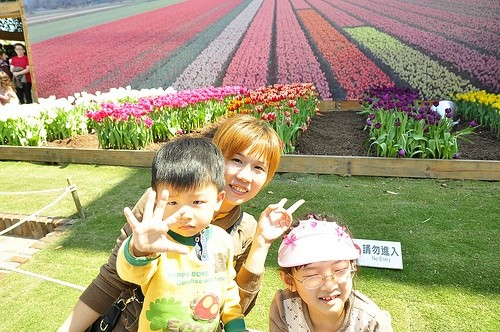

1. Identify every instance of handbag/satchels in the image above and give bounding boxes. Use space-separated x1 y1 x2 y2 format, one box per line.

84 299 126 332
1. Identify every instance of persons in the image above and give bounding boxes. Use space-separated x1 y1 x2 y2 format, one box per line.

0 42 33 105
116 137 248 332
56 113 305 332
268 213 394 332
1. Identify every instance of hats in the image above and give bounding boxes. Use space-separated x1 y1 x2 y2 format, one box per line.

278 219 360 267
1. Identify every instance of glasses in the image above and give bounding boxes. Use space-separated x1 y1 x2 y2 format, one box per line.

287 261 355 290
